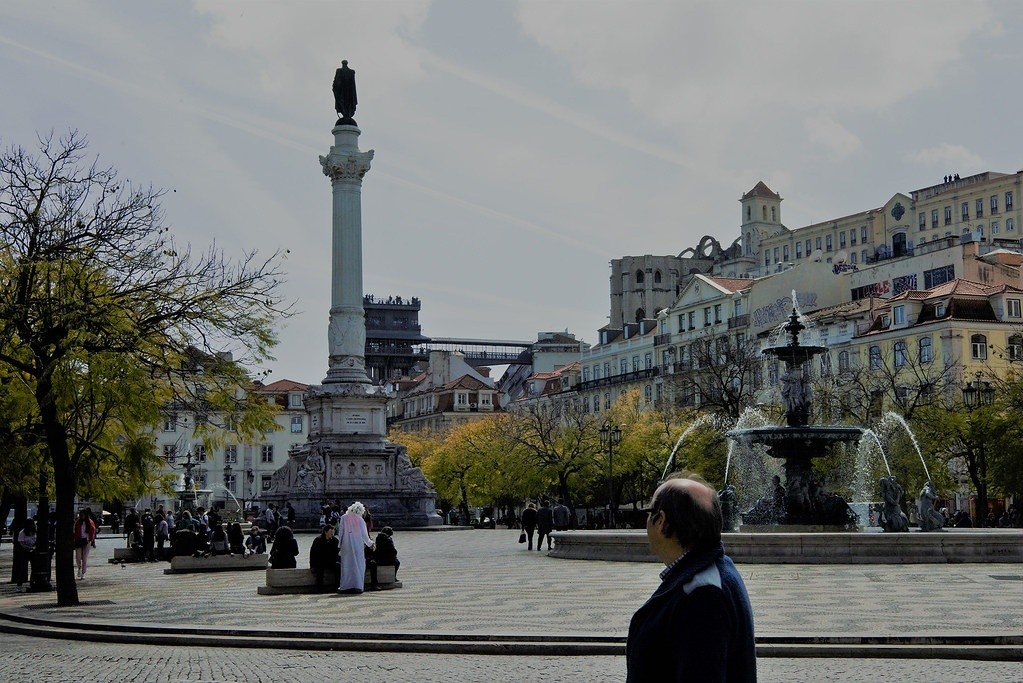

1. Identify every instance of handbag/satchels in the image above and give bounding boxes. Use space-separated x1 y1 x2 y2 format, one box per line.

518 534 526 543
75 538 87 548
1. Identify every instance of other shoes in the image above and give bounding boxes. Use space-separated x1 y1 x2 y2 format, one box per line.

537 549 541 551
371 586 381 590
77 574 85 580
548 548 553 550
267 541 273 544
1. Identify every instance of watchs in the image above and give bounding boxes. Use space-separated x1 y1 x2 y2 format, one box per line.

111 512 120 534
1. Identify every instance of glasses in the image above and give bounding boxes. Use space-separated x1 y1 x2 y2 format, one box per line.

251 530 256 531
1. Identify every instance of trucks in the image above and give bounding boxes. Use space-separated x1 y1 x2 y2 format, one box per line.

6 502 56 527
74 503 102 525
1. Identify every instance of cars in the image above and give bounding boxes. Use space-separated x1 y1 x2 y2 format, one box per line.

470 516 490 528
435 509 446 524
102 510 119 524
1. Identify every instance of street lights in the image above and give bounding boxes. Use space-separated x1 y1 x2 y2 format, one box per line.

963 381 996 526
224 464 232 510
599 419 623 529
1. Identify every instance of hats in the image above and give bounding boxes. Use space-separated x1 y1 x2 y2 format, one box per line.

528 504 534 508
556 499 564 504
382 526 393 535
366 522 373 529
544 500 550 506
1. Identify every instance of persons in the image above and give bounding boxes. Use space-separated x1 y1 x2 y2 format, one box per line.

938 504 1018 527
919 481 942 531
396 443 434 492
878 475 909 532
496 516 503 525
553 498 570 531
536 500 552 551
297 444 325 490
624 471 757 683
3 504 101 591
772 473 785 510
123 496 399 593
521 502 537 550
910 507 922 526
717 482 739 533
